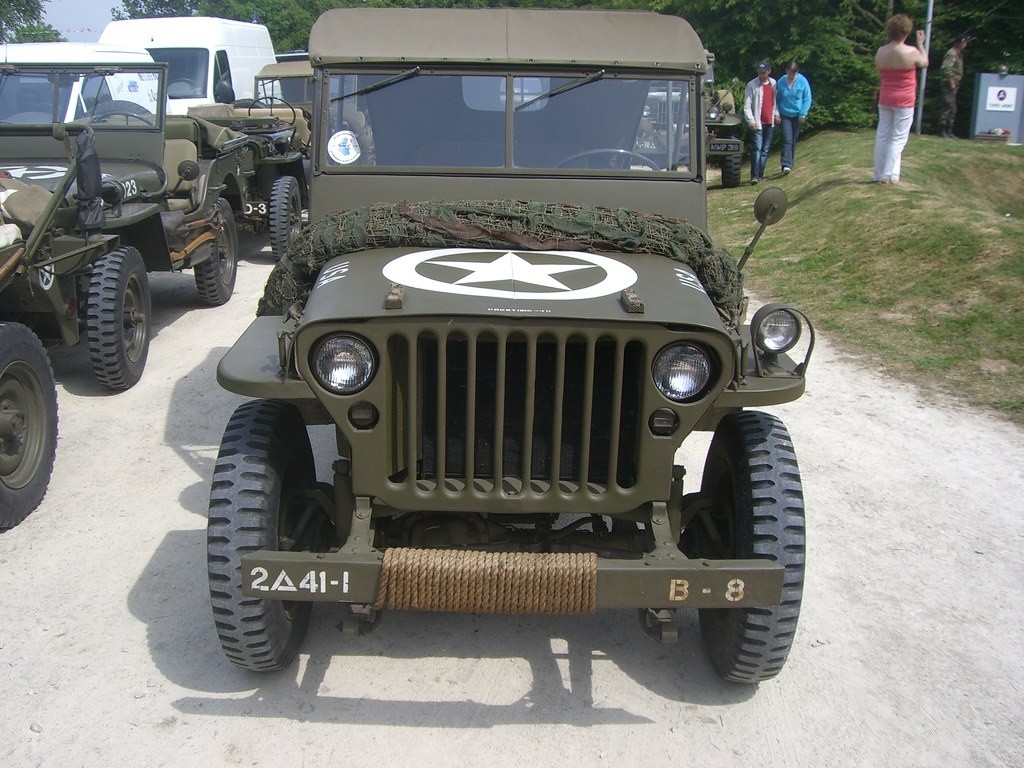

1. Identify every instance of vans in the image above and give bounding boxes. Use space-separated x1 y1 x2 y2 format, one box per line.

99 15 282 118
0 42 173 123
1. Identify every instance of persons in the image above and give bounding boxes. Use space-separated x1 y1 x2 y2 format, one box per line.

874 14 928 185
938 36 968 140
775 59 812 174
744 63 781 186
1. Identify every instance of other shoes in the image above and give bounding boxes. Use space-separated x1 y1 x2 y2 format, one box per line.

938 128 958 138
784 166 791 173
752 176 764 184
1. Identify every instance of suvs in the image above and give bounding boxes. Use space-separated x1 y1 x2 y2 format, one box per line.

0 57 237 524
204 7 815 683
627 46 744 188
188 59 376 262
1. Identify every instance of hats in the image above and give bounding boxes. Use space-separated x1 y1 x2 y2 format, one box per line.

998 64 1009 75
756 62 770 72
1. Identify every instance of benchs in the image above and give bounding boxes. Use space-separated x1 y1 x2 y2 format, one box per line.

187 104 309 143
4 112 202 211
